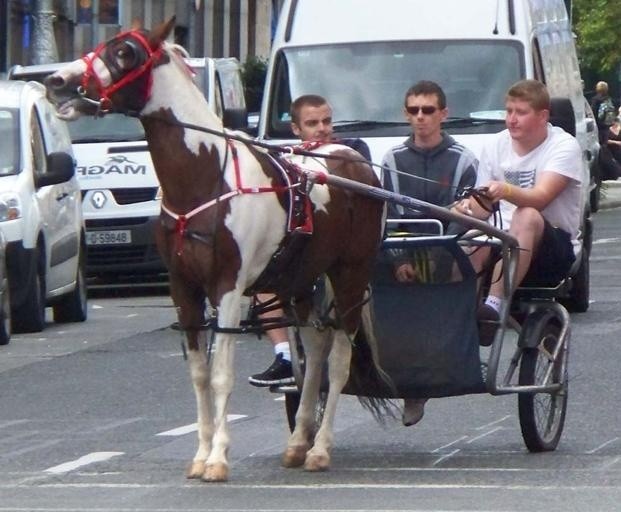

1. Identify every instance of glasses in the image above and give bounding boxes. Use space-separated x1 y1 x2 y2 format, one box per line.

405 105 441 114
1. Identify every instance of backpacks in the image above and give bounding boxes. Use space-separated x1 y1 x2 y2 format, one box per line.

591 95 616 128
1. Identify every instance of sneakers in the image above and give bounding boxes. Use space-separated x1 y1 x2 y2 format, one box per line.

476 304 500 342
402 396 429 427
246 352 301 389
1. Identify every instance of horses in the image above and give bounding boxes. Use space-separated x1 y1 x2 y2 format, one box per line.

42 13 386 484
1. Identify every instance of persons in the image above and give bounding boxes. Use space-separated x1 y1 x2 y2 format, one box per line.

379 80 479 426
595 81 615 120
607 117 621 160
245 95 374 387
450 79 584 346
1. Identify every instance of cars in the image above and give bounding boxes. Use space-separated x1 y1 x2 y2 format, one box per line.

583 94 602 211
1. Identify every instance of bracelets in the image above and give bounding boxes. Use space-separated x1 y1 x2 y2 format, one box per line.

506 182 513 201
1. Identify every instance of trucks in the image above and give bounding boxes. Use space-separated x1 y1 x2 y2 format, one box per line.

246 0 597 312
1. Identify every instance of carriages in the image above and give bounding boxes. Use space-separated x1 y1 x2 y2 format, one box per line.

36 9 599 489
